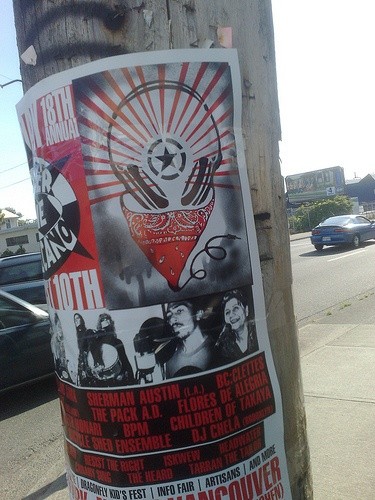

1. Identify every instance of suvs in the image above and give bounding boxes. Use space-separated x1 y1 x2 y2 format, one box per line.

0 251 47 305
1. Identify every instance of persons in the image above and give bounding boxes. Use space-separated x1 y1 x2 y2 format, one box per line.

49 309 135 389
159 299 219 381
212 289 260 364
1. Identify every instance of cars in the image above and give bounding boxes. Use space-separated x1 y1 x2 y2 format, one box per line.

310 215 375 250
0 287 54 398
